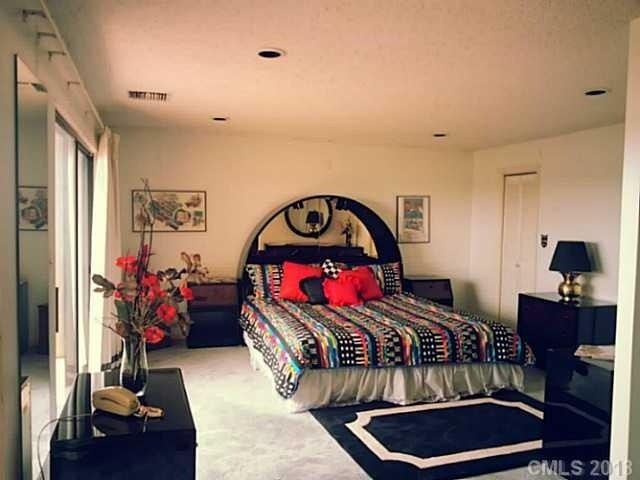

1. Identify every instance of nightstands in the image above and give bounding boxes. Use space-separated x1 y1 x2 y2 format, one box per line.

404 275 454 309
187 278 240 349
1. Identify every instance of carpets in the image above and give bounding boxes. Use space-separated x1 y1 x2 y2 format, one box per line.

308 390 543 480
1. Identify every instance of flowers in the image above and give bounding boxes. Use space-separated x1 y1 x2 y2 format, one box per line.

91 177 208 343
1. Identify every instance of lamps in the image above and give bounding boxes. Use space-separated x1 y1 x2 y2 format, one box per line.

549 241 593 302
306 210 324 233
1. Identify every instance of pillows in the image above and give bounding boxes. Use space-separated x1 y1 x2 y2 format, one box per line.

338 267 383 301
322 278 363 307
334 261 403 296
299 277 328 305
280 262 323 303
322 259 344 280
246 264 321 302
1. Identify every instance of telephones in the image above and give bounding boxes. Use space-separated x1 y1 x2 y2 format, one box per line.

93 386 165 416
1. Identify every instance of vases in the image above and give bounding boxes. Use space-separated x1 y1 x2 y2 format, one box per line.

120 333 149 397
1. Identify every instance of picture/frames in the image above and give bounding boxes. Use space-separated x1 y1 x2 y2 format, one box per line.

131 189 207 233
18 185 49 231
397 195 431 245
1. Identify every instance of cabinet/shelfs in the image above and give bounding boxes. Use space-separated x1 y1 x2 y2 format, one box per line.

544 341 614 480
517 292 618 370
52 367 198 480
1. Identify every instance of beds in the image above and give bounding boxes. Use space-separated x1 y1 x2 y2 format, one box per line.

236 195 536 413
